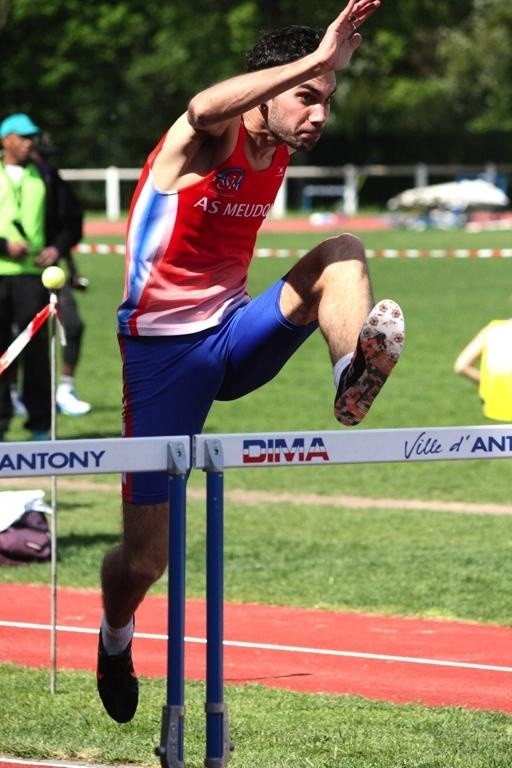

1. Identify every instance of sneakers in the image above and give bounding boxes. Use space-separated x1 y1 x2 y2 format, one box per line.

55 383 92 416
334 298 405 426
97 613 138 722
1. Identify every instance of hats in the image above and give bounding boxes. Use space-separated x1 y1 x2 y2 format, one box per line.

0 112 41 139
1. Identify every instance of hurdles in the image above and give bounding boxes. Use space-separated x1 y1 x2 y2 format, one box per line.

0 425 512 768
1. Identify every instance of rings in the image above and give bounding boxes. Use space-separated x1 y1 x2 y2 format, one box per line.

352 22 357 30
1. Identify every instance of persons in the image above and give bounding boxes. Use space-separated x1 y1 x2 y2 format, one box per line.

0 111 84 441
455 316 512 423
96 1 409 723
9 145 94 416
